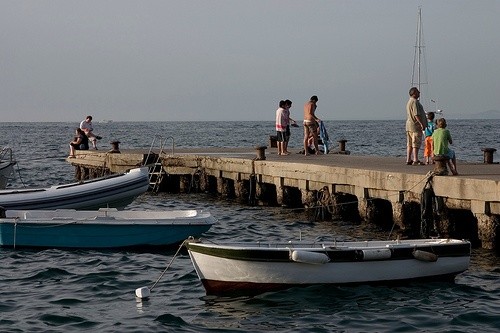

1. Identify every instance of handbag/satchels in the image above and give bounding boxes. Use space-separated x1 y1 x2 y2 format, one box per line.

319 121 328 140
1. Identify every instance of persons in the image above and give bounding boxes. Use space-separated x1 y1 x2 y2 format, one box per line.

303 95 321 155
80 116 102 150
276 100 291 156
431 117 458 176
68 127 89 158
405 87 428 165
284 99 296 154
424 112 437 166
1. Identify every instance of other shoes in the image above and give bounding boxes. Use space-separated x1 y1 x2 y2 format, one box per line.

96 136 103 139
412 161 423 165
406 160 412 165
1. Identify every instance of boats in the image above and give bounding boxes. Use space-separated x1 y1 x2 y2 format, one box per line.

0 209 218 250
0 167 151 212
182 237 473 299
0 146 18 189
